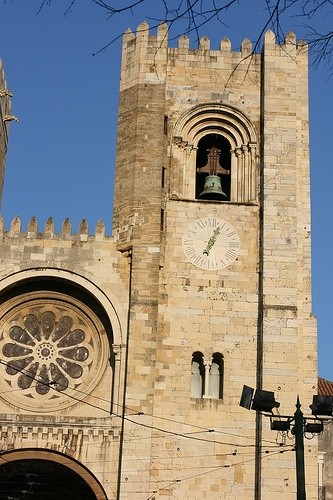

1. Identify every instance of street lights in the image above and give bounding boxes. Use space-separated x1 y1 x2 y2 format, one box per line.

239 384 333 500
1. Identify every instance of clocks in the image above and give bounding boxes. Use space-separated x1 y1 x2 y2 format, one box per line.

181 217 241 271
